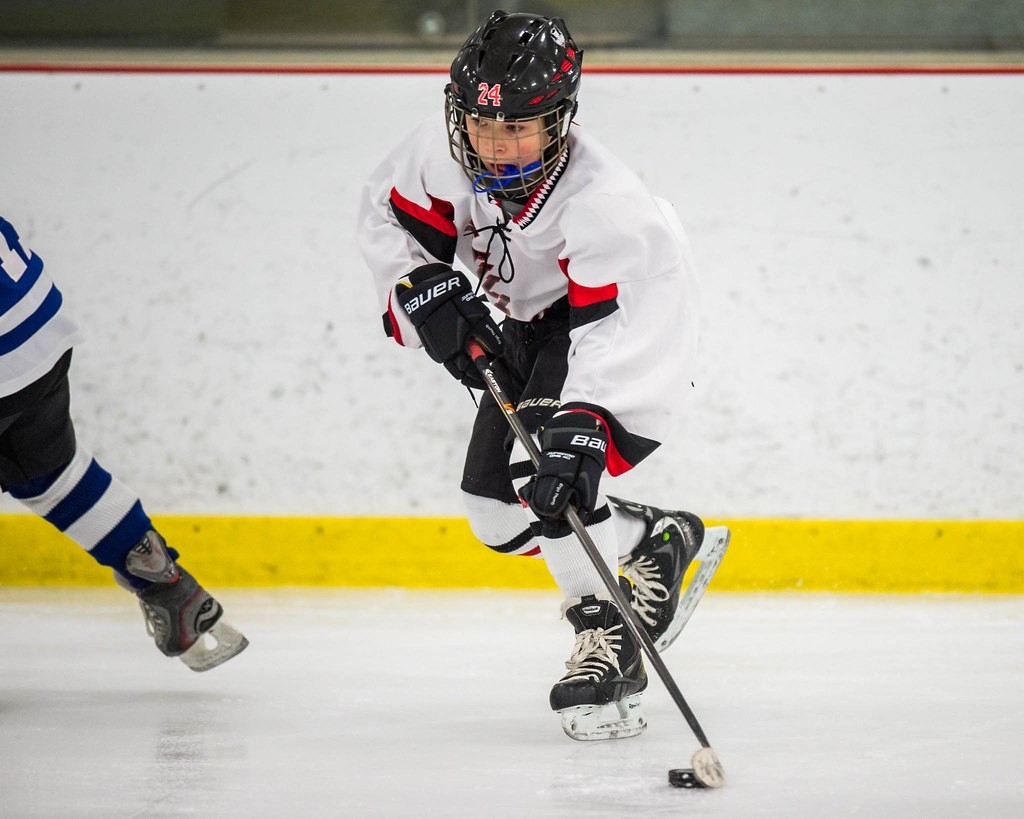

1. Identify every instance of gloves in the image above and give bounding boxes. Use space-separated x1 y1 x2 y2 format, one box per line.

518 413 608 539
398 271 508 390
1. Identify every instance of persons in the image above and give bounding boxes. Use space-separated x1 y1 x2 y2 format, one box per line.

355 8 732 741
0 216 250 672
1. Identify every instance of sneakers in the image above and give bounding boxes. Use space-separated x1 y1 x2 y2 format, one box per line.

115 531 248 671
550 575 648 738
606 494 729 653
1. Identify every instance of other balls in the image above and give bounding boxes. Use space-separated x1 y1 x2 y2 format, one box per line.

670 768 704 788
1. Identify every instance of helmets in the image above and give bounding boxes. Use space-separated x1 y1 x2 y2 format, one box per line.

443 9 583 199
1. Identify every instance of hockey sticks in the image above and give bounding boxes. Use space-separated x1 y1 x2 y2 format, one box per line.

469 342 727 789
